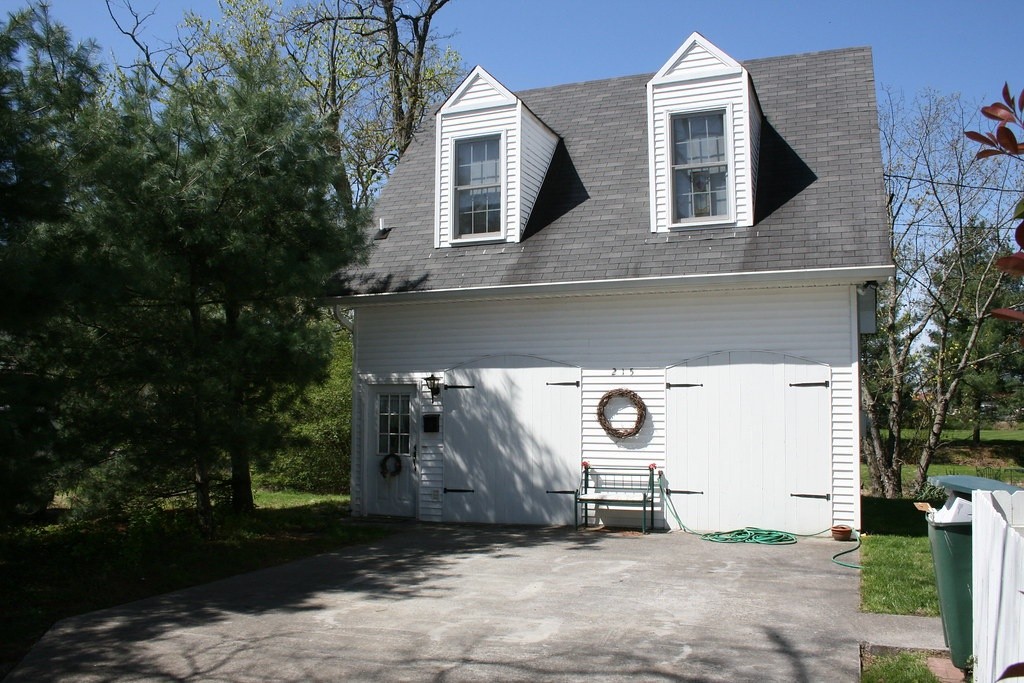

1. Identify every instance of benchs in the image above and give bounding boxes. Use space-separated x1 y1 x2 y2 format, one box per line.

575 461 656 534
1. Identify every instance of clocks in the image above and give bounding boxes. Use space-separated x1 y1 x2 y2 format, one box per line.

690 171 710 216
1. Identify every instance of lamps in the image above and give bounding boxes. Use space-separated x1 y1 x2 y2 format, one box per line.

423 374 441 404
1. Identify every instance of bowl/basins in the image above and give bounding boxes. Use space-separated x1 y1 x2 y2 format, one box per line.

832 527 852 541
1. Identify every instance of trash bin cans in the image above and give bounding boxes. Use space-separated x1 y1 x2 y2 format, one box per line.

925 474 1024 670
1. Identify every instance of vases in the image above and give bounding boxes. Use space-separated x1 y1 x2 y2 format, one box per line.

832 527 852 541
